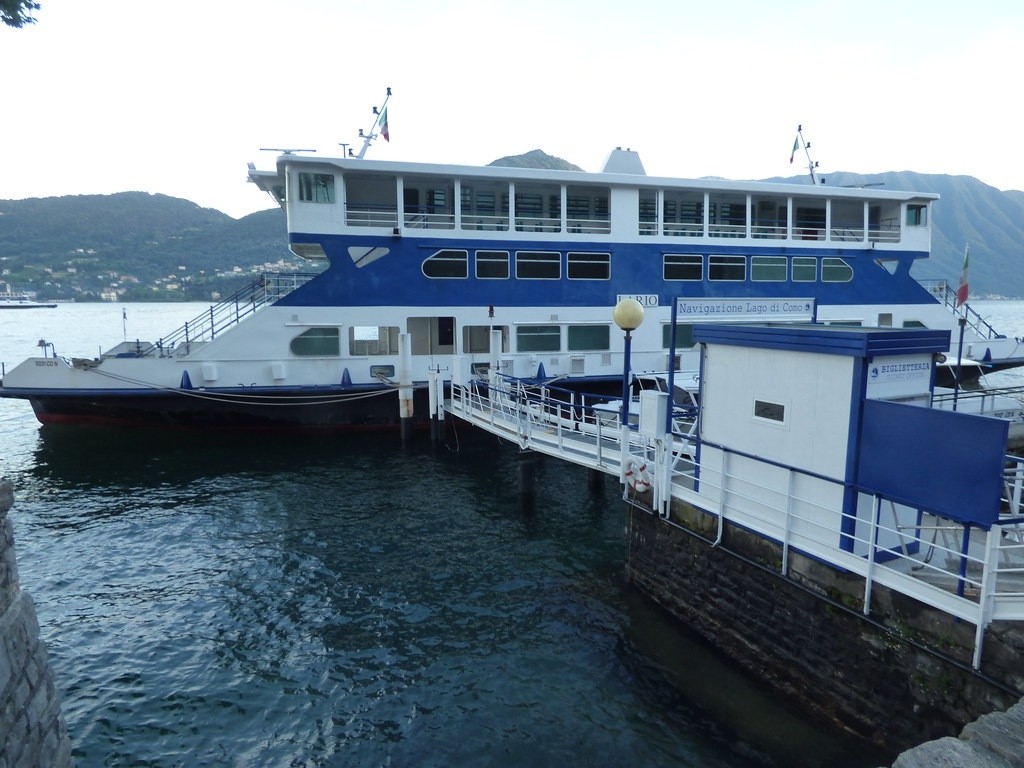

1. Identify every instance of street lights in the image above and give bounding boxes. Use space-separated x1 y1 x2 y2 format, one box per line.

614 297 643 482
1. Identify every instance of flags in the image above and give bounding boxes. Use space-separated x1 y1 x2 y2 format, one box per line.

379 106 389 142
958 248 969 306
790 136 801 164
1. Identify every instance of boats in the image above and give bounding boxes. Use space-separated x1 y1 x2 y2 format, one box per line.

934 352 1024 449
593 371 701 443
0 146 1024 435
